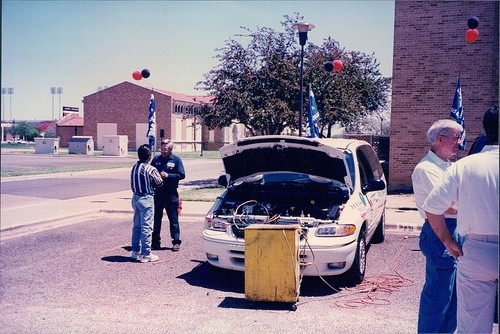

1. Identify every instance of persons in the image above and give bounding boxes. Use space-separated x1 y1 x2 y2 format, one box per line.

421 106 500 334
150 139 185 251
411 119 468 334
130 144 164 262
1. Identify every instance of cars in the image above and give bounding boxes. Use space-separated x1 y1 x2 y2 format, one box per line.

201 134 387 284
7 137 27 144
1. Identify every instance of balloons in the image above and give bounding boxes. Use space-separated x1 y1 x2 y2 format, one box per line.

468 16 478 29
132 70 142 80
333 60 342 71
141 68 151 78
324 62 334 72
466 29 478 43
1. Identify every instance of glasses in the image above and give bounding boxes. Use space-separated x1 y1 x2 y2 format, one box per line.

442 135 462 141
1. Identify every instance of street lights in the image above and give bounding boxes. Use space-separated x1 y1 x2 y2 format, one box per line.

290 21 315 136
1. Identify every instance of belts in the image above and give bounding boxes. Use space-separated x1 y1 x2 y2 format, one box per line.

135 192 153 196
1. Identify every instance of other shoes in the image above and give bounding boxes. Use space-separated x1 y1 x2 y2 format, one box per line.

140 252 159 262
172 244 180 251
131 251 142 260
151 244 161 250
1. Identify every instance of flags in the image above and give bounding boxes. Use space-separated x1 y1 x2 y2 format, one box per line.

450 81 466 151
145 93 156 153
306 84 320 139
